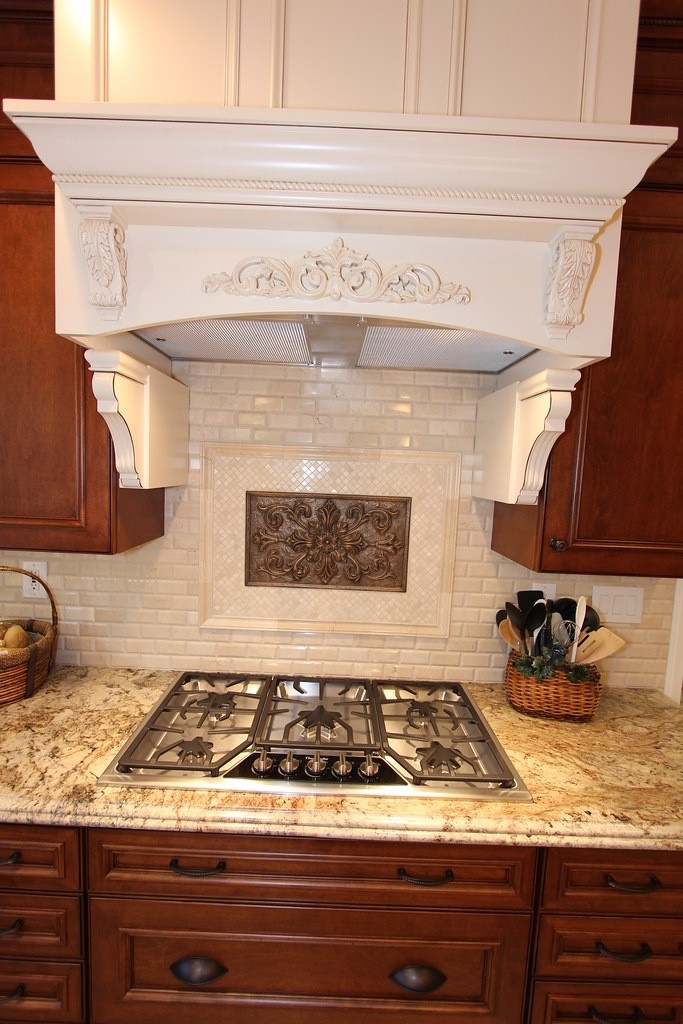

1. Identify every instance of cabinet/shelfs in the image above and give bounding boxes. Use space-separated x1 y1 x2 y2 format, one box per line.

87 827 538 1023
530 848 682 1024
0 822 84 1024
1 0 167 556
491 1 683 578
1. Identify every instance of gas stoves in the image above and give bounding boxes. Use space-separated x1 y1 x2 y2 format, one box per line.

96 668 533 803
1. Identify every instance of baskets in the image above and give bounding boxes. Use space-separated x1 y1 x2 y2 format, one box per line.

506 649 602 723
0 566 59 707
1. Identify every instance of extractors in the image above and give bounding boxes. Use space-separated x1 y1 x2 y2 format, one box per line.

128 312 541 374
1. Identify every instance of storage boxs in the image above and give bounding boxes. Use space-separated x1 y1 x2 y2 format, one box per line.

0 564 59 707
504 648 602 723
